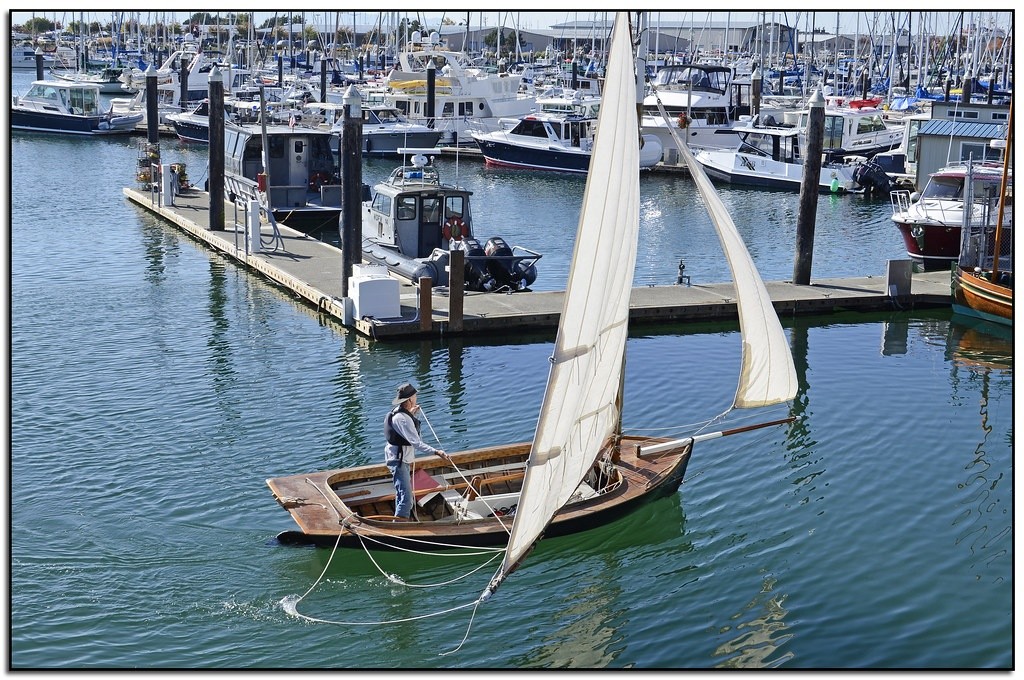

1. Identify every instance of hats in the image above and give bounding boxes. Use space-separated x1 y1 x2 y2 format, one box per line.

392 383 417 404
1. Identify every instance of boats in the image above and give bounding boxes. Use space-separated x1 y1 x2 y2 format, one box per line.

326 102 449 157
12 74 143 135
164 97 303 142
207 106 372 212
642 63 736 119
109 73 181 125
889 123 1012 264
695 114 868 192
464 108 663 172
337 148 543 293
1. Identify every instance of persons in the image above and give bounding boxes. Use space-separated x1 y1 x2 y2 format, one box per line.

383 383 449 522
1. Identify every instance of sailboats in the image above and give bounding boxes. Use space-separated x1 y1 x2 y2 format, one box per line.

953 327 1012 439
12 12 1012 114
950 93 1013 325
267 12 798 601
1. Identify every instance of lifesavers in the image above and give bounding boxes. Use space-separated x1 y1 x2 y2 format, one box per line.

443 215 469 242
